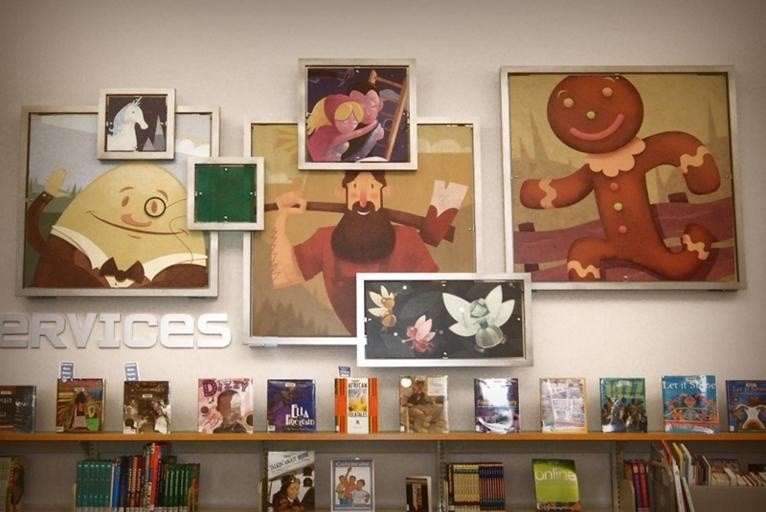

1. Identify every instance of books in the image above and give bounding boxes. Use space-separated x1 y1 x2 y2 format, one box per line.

530 458 583 512
0 384 37 433
266 379 316 432
601 379 647 434
122 382 172 436
406 476 432 512
267 450 315 512
197 378 255 434
1 455 26 512
623 442 766 512
473 377 519 435
663 374 766 434
445 463 506 512
56 378 105 434
400 374 448 435
334 376 378 435
540 377 587 434
73 442 200 512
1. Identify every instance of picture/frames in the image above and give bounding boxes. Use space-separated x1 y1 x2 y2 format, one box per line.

15 102 220 297
98 86 175 162
355 272 534 369
499 62 748 291
188 157 265 231
297 56 419 170
244 117 482 346
